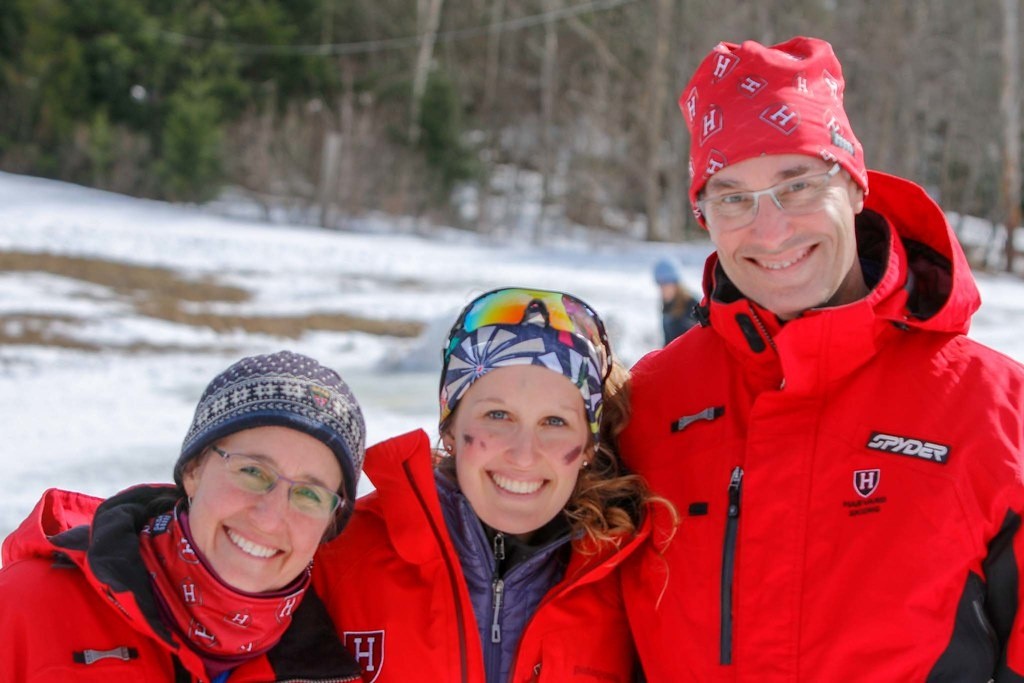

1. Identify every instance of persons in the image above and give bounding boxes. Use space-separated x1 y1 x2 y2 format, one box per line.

617 35 1024 683
653 256 700 346
311 287 648 682
0 350 369 683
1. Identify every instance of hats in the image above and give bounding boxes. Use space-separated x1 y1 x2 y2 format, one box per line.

655 259 679 284
678 37 868 229
174 351 366 544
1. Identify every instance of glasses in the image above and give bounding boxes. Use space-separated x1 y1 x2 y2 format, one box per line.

445 288 608 377
210 445 345 518
697 162 841 230
